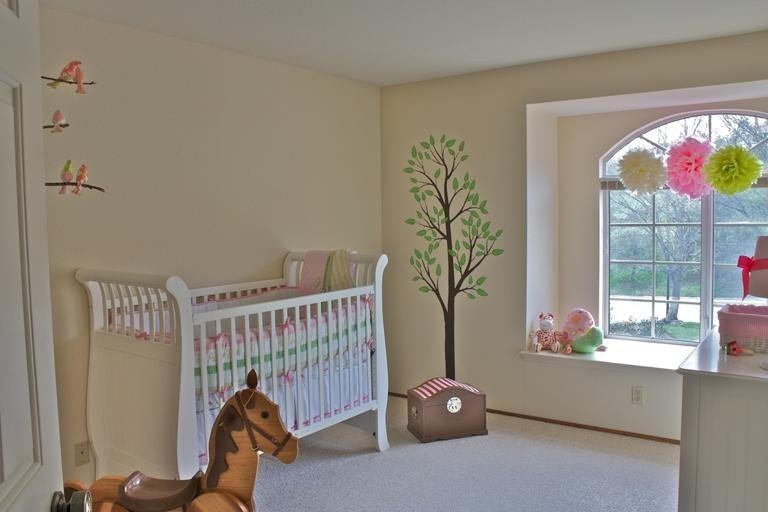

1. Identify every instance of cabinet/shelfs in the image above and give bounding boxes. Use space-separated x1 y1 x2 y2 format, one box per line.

676 326 768 512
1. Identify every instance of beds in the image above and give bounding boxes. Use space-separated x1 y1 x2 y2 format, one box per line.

74 251 390 483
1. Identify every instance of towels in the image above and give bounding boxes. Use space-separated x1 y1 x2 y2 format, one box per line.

299 248 358 302
